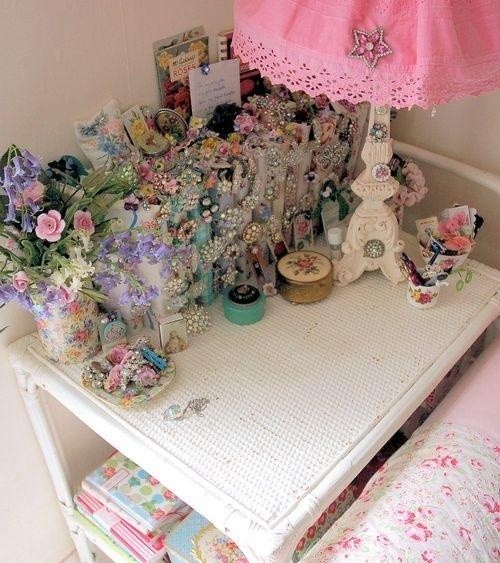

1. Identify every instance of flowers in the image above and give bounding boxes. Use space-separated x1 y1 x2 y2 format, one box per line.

0 144 147 315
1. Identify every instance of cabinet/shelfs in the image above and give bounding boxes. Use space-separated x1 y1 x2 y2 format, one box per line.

6 209 500 563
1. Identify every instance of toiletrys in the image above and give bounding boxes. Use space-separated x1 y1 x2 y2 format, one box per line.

221 279 267 326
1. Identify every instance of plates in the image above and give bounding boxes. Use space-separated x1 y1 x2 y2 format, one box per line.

81 343 177 408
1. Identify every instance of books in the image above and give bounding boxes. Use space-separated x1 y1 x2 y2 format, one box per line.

218 29 270 98
153 35 210 121
69 453 191 563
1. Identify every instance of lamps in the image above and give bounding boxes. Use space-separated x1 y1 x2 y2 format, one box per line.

232 0 500 287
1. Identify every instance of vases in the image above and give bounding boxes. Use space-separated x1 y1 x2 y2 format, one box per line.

23 275 102 364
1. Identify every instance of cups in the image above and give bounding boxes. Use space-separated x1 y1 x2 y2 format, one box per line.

418 243 470 272
407 268 440 309
